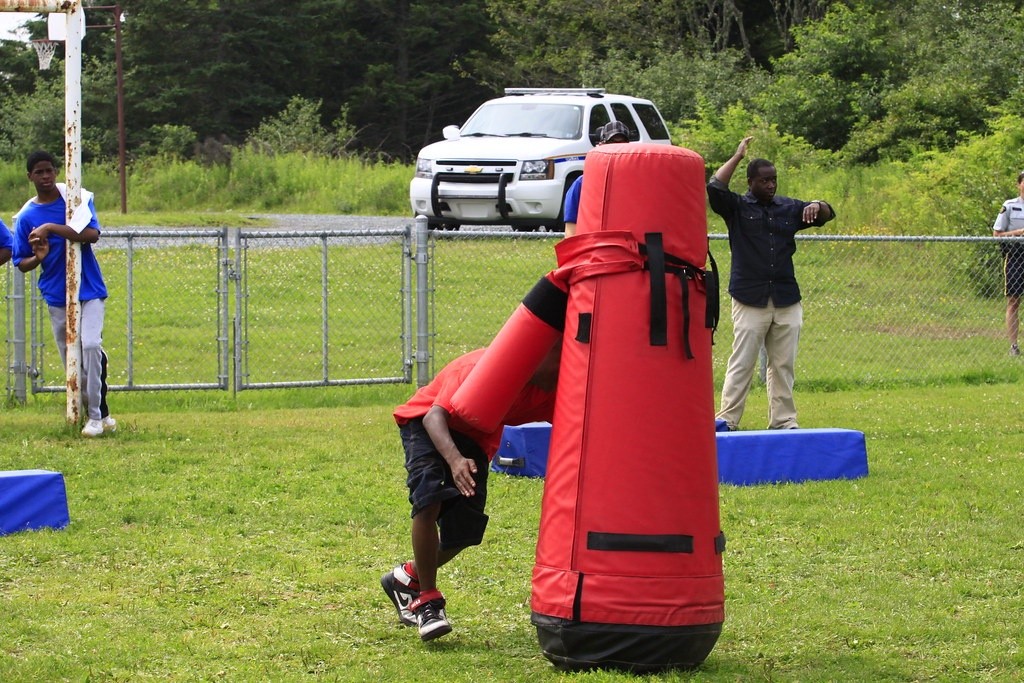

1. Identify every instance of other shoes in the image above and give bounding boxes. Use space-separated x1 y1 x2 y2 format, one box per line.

81 418 104 435
99 417 116 430
1009 345 1019 356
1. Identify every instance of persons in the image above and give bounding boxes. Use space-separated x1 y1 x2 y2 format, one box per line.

11 153 117 439
706 137 835 432
561 122 634 240
993 172 1024 358
380 337 563 641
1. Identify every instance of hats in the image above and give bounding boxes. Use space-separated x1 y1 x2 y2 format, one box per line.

603 120 629 142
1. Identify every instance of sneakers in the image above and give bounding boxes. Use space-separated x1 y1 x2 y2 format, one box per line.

381 562 452 641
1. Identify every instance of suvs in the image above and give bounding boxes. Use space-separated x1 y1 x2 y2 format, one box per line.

409 85 674 237
